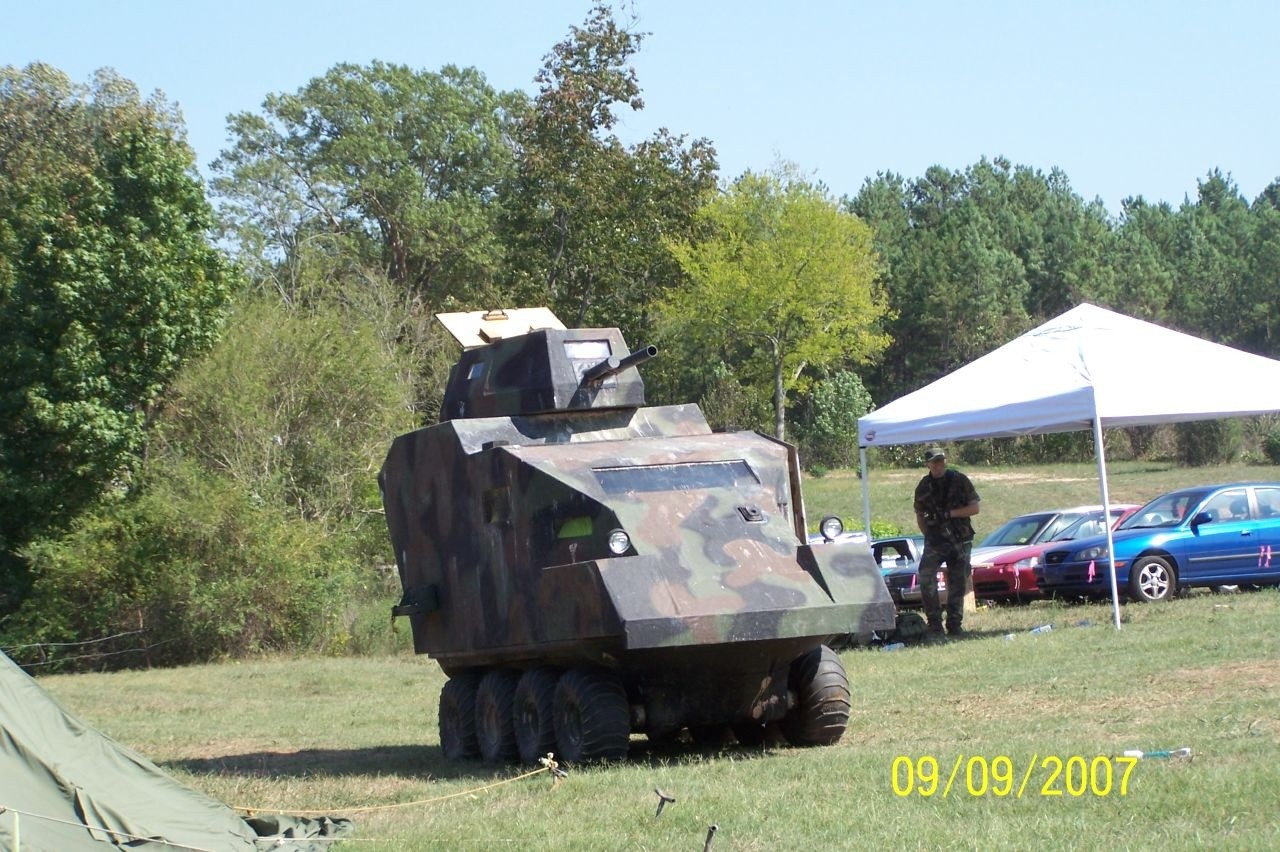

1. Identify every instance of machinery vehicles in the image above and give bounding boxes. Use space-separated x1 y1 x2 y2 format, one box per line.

376 305 898 767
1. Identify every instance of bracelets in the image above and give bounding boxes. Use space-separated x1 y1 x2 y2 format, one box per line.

947 510 951 520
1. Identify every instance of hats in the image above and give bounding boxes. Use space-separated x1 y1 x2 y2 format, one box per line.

925 447 945 462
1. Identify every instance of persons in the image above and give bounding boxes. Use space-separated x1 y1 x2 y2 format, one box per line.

911 446 981 637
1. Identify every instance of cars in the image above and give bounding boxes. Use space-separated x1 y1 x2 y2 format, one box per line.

1040 480 1280 605
803 502 1186 611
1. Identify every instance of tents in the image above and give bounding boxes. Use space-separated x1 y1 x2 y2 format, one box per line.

856 302 1280 633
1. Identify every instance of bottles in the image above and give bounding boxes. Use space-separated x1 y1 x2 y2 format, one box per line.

1002 633 1016 641
1028 625 1054 634
881 642 905 652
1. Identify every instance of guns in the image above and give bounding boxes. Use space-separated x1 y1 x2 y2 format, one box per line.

917 491 966 562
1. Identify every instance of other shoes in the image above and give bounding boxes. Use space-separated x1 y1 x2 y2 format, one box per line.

946 624 964 635
926 623 946 636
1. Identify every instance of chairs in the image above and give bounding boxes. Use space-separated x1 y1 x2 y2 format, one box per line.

1229 500 1249 520
